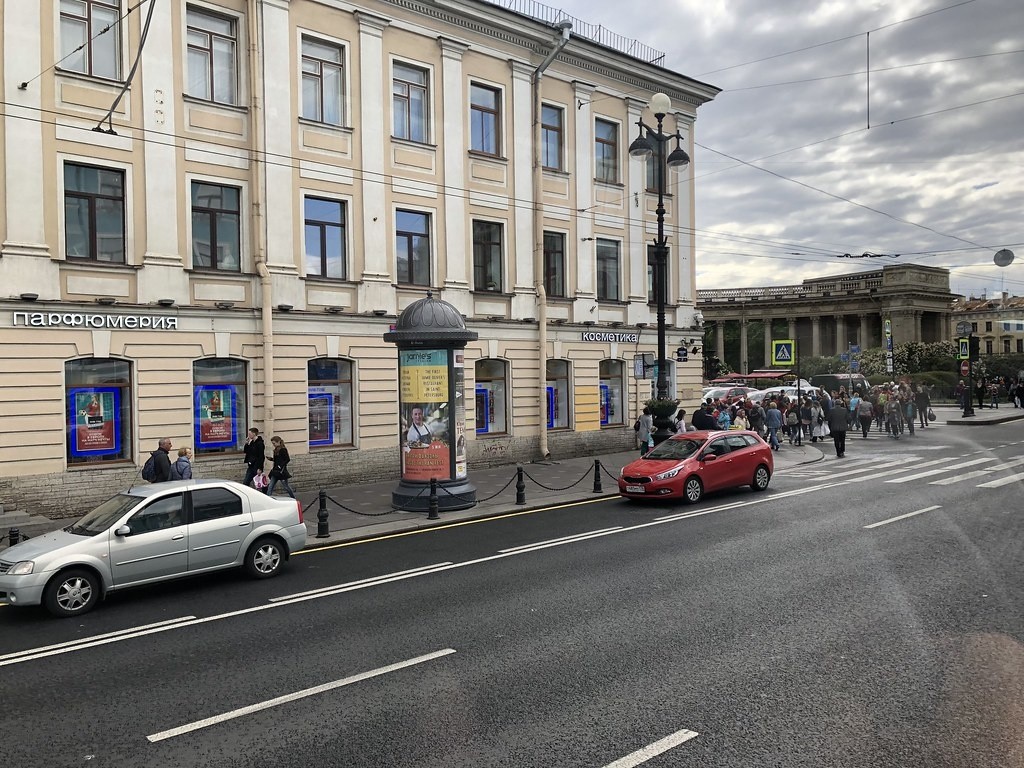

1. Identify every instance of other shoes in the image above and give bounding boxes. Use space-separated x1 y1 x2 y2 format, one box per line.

789 439 792 444
839 452 845 457
920 425 924 428
775 445 779 451
771 447 774 449
925 422 928 426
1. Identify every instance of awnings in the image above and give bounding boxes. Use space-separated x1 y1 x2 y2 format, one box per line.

747 370 791 378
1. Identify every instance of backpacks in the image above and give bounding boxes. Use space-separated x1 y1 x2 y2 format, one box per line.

669 418 680 432
788 409 798 425
142 451 166 482
750 407 761 420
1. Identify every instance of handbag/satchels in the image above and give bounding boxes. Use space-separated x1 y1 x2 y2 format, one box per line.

817 409 823 425
634 415 641 431
253 474 268 489
928 409 936 421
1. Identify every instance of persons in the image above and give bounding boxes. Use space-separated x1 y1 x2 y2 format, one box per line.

168 447 192 481
82 395 100 424
150 438 172 484
266 436 296 499
673 409 686 435
990 384 998 409
206 391 221 418
764 402 782 451
827 399 852 458
1010 380 1024 410
407 405 432 446
954 380 966 410
243 428 268 495
637 407 656 456
975 380 988 409
692 380 935 446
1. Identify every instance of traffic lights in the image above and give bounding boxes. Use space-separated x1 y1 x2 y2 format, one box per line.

705 357 722 380
953 337 963 355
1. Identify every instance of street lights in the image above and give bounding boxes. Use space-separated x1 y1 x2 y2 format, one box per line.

622 89 691 455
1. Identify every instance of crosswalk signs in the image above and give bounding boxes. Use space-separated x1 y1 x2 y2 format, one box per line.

959 339 970 359
771 341 796 366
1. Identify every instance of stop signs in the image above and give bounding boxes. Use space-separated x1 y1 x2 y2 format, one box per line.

960 360 970 377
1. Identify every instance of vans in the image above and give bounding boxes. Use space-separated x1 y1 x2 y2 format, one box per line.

811 374 871 394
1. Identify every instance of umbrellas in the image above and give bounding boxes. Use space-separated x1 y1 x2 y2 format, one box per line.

713 373 745 382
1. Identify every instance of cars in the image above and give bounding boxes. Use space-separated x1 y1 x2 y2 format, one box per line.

616 430 773 508
0 479 308 618
1008 382 1024 410
702 384 831 411
